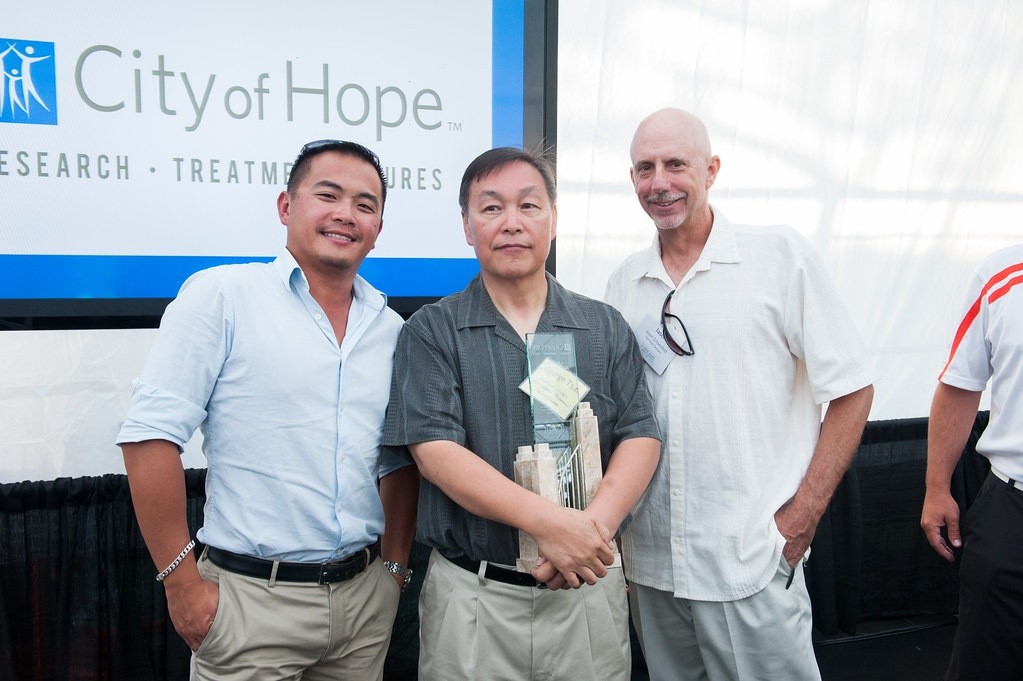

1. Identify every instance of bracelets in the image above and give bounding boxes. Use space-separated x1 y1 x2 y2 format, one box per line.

155 540 196 582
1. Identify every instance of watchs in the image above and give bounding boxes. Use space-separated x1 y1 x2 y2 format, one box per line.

382 560 413 593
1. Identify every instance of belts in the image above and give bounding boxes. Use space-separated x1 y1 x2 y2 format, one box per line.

206 544 379 582
438 547 538 587
990 465 1023 491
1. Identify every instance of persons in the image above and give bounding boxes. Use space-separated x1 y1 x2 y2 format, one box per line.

116 139 407 681
601 108 874 681
920 239 1023 680
381 146 661 680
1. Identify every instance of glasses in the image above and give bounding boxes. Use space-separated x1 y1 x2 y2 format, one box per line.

293 139 381 167
660 289 695 356
